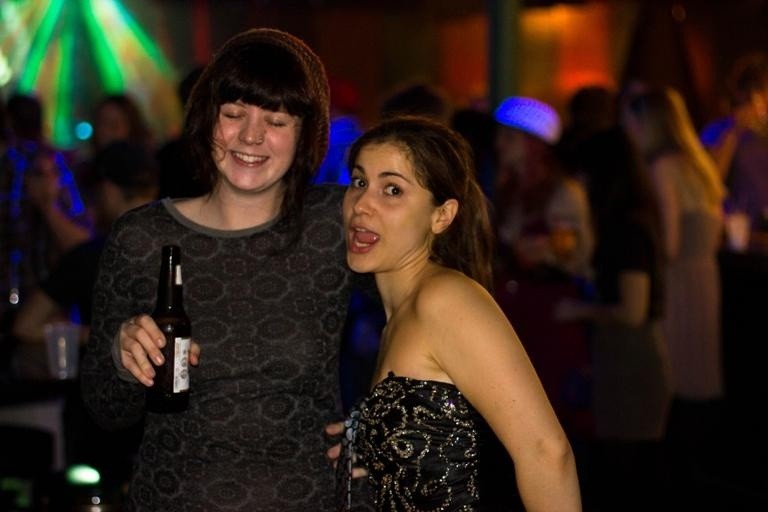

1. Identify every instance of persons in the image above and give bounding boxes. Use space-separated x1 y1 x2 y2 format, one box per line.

342 116 582 512
78 28 348 512
1 65 214 438
362 81 767 438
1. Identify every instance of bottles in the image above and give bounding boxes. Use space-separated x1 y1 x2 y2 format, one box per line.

150 244 191 408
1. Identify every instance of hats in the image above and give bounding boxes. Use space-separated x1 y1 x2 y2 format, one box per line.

493 95 563 144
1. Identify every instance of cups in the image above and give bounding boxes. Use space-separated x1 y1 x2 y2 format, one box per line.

44 323 81 379
